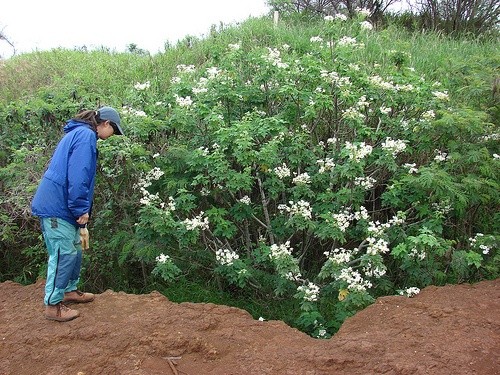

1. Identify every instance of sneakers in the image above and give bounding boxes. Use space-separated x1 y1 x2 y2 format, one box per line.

45 303 80 322
64 289 94 304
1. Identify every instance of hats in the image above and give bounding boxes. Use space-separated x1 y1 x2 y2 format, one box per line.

96 106 125 136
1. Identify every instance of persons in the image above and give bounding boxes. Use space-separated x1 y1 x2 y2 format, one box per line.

31 106 125 322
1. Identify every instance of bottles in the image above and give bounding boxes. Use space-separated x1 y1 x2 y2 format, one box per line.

80 223 90 251
